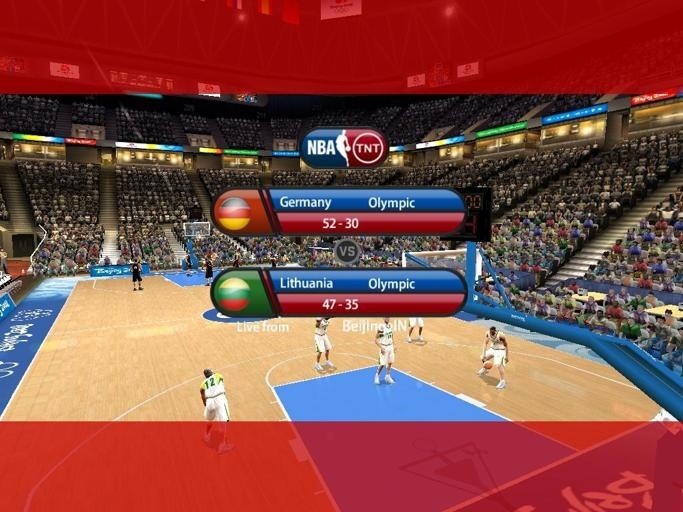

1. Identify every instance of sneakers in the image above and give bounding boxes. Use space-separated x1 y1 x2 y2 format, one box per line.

204 433 234 454
375 376 394 384
479 367 506 389
314 360 334 370
408 336 423 343
